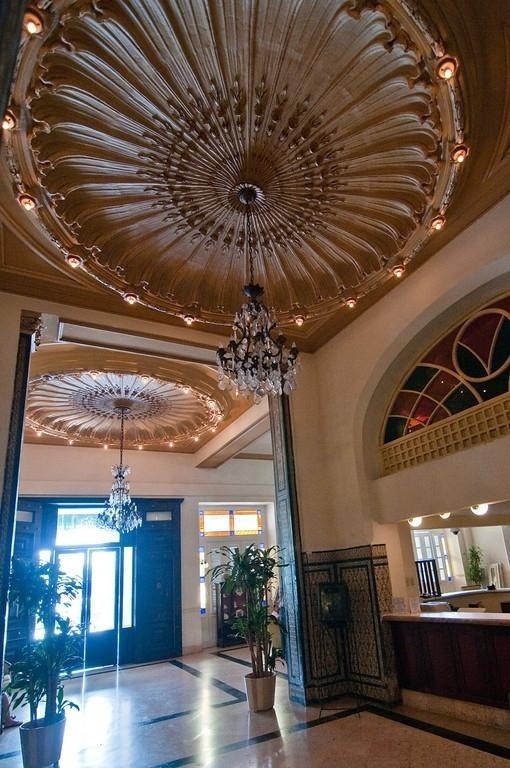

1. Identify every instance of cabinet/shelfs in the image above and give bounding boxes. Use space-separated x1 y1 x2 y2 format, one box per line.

215 582 257 648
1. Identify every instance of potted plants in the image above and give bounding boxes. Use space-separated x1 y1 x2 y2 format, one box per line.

203 545 289 714
1 553 92 768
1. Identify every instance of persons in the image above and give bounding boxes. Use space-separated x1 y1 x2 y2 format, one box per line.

271 604 281 619
2 691 23 727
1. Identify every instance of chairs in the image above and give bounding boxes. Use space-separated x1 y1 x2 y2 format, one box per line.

266 615 285 656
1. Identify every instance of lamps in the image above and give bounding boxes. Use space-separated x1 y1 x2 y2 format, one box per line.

215 189 301 405
96 398 142 535
